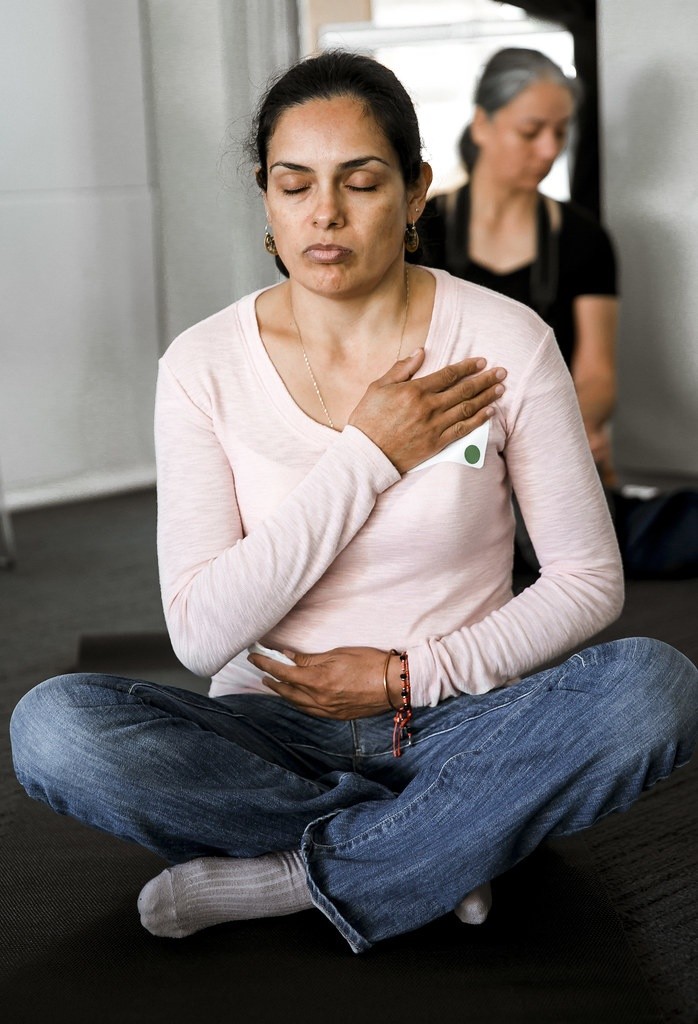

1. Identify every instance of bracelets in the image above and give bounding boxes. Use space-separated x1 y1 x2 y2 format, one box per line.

383 647 413 758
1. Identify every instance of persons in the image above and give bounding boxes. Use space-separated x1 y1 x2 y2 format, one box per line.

11 52 698 938
406 47 620 493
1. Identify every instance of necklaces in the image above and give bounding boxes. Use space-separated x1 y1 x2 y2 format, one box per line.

287 263 410 433
